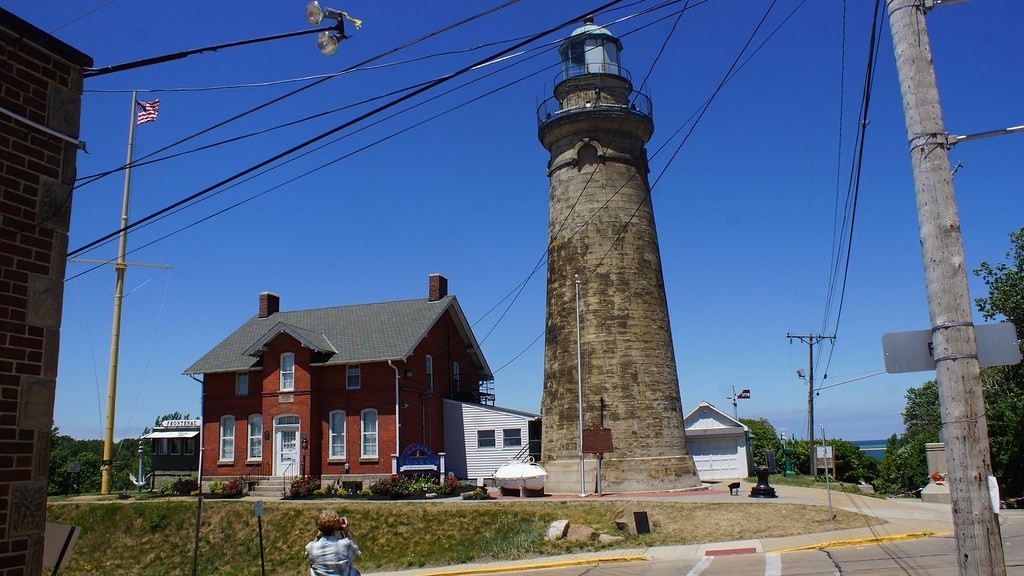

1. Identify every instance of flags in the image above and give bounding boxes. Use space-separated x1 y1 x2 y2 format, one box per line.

135 99 160 127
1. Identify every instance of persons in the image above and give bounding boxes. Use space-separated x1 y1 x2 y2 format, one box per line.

71 480 76 494
305 509 361 576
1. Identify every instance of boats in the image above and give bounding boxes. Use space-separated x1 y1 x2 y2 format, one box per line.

493 460 549 490
